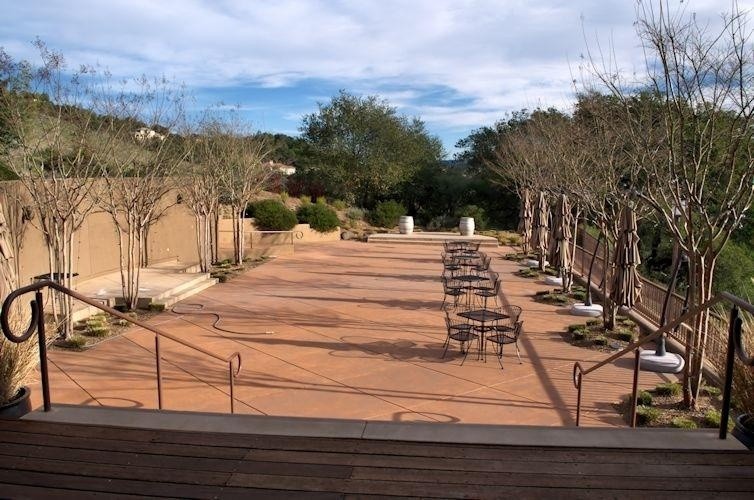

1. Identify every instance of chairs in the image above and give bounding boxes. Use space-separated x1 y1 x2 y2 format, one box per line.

440 240 524 372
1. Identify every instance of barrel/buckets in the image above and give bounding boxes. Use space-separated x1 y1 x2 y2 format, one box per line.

399 215 413 233
459 217 474 236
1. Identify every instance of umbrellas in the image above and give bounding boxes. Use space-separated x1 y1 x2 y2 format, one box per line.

529 190 553 272
517 187 533 255
606 200 644 332
552 194 575 292
